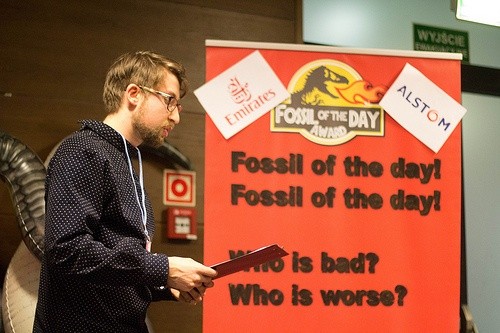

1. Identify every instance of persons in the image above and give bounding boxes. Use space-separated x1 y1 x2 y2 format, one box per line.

32 50 219 333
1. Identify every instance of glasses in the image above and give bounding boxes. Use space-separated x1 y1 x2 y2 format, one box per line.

139 85 182 114
